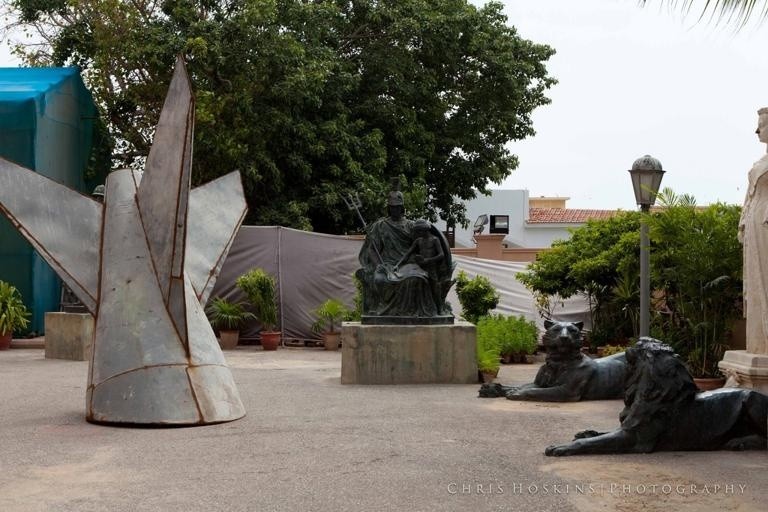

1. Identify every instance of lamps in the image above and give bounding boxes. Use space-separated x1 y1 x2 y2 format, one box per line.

474 214 509 235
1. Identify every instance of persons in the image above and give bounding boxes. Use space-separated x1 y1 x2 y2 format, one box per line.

355 190 438 315
394 218 446 316
736 106 768 353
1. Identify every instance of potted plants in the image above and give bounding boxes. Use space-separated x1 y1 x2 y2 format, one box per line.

204 267 281 350
307 298 351 350
1 282 31 350
678 254 731 393
476 312 538 383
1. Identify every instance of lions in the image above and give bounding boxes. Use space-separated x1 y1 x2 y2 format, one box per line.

545 337 768 456
478 319 630 402
